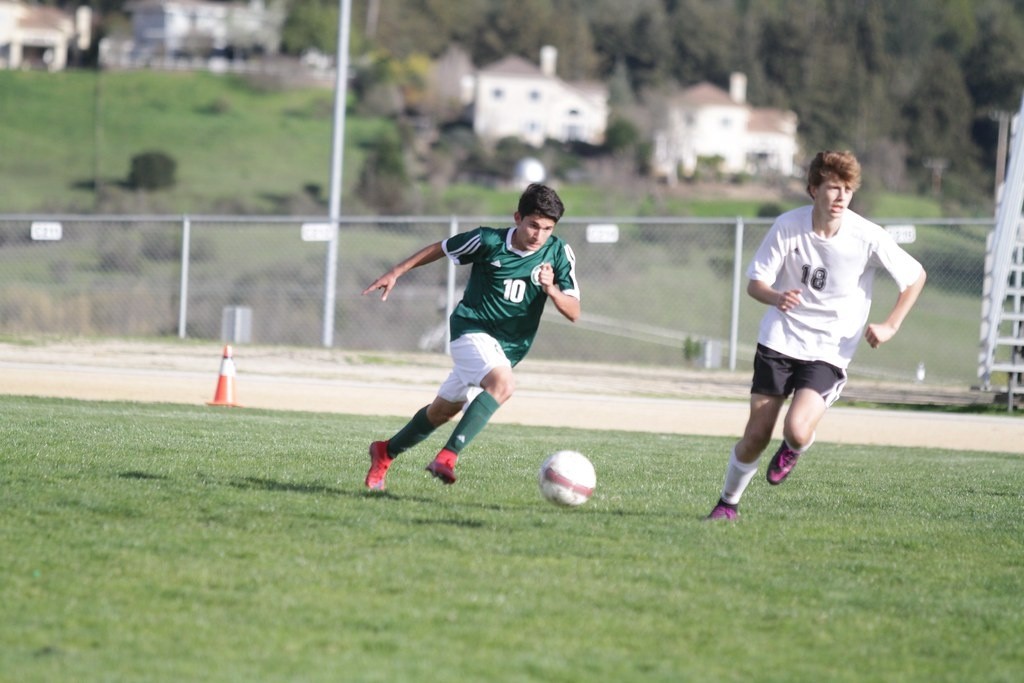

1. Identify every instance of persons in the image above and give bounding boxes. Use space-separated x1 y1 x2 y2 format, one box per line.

361 183 579 494
707 148 927 519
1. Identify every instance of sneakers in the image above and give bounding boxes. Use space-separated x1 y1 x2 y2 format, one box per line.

425 461 456 485
767 439 801 485
706 506 736 520
366 440 393 491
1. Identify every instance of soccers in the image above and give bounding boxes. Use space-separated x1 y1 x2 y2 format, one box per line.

536 450 597 508
